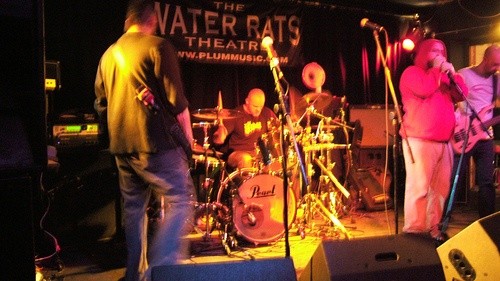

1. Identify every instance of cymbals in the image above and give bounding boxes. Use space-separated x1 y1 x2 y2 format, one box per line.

192 109 247 120
308 125 344 130
293 92 332 118
190 143 224 156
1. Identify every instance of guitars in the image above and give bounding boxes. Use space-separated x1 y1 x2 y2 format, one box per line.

448 102 500 155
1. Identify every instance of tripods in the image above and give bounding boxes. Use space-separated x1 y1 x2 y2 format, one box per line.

294 108 357 240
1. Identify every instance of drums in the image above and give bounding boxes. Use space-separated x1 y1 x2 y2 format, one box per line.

255 130 296 174
189 154 227 208
215 166 298 246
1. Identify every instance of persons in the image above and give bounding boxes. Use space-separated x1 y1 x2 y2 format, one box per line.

95 0 195 281
398 39 470 234
443 45 500 222
214 88 282 173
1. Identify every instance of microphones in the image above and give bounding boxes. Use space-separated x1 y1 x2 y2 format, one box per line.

262 36 283 79
242 202 256 226
360 18 385 32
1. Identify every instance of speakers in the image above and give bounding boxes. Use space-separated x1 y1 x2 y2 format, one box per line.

137 257 298 281
348 168 391 211
350 148 387 170
445 153 470 206
435 210 500 281
297 231 446 281
0 0 125 281
350 105 401 148
